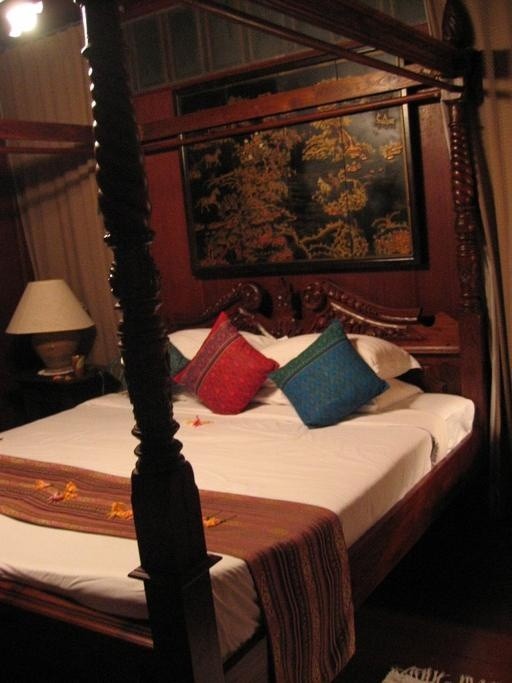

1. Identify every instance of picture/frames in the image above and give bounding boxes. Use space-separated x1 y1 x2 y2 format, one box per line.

173 47 428 277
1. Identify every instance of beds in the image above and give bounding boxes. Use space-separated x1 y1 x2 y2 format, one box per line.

0 1 492 682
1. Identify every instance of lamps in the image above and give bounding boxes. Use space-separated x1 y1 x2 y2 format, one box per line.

5 278 95 374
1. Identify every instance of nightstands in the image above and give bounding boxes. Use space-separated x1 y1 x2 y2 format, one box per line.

19 362 113 424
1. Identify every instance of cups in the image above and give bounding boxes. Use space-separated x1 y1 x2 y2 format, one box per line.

71 356 86 377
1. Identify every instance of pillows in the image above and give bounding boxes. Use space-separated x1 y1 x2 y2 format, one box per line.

250 377 424 411
264 320 390 428
260 331 422 386
172 311 280 415
166 328 275 360
